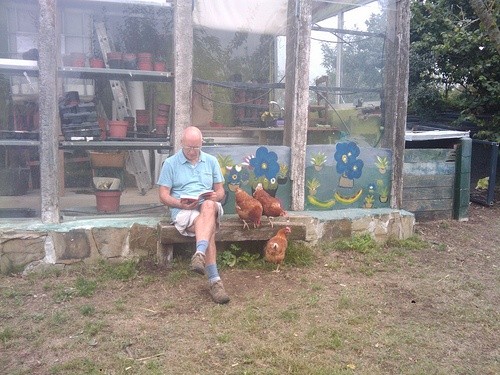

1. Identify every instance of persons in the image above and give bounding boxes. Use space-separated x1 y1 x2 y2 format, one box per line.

157 126 230 304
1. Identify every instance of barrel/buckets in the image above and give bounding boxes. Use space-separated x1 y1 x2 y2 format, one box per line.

109 103 171 139
62 51 165 72
95 191 121 214
0 167 30 196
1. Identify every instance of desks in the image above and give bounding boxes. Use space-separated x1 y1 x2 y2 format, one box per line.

242 126 339 146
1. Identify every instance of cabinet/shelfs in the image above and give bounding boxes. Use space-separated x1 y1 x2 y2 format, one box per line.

0 59 174 223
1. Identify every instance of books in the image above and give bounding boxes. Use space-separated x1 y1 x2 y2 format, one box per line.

180 190 214 208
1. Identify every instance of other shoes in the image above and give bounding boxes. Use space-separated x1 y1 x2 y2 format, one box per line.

206 278 230 304
191 252 206 275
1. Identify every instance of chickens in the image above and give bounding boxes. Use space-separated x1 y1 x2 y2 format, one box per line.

263 226 292 274
234 186 263 229
251 183 287 229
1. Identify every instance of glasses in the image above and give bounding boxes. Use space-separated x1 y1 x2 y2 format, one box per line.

182 144 202 152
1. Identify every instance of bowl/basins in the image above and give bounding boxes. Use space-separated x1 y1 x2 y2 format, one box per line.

90 153 125 168
92 176 121 190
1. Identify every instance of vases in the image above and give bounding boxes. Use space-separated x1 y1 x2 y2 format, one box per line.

64 52 167 71
87 149 126 213
62 92 170 140
